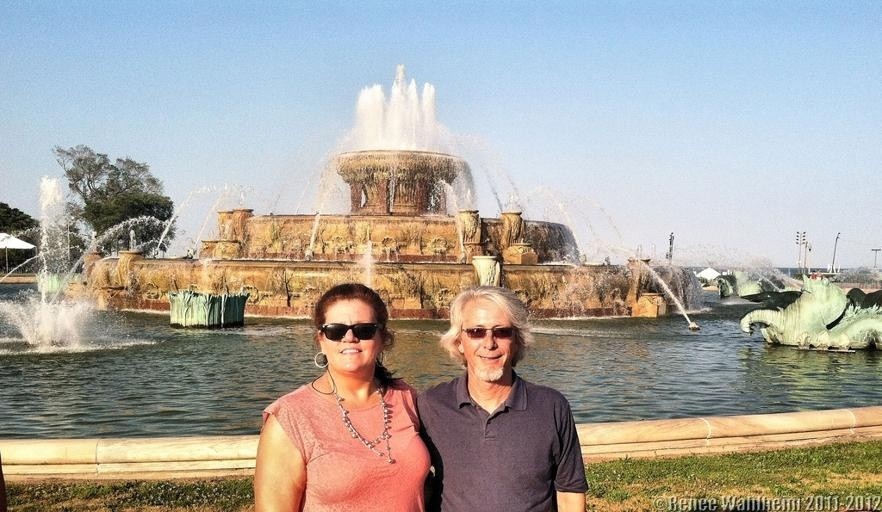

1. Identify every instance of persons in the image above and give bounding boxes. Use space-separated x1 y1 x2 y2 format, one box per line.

416 284 589 512
811 271 816 279
254 282 432 512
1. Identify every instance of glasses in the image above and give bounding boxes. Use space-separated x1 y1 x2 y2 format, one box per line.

318 323 379 340
463 328 514 338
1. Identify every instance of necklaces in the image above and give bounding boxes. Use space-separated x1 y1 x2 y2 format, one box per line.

328 377 394 464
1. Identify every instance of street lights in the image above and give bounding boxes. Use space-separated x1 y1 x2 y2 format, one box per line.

871 248 881 269
794 231 806 270
